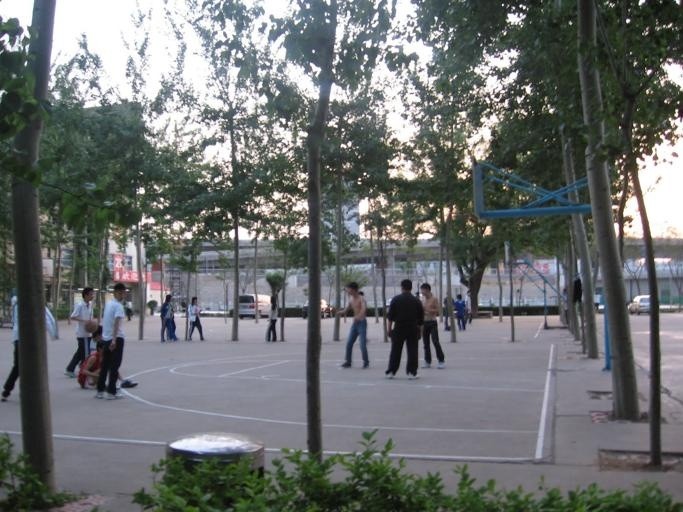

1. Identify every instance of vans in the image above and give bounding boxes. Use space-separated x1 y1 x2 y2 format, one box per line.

238 294 273 321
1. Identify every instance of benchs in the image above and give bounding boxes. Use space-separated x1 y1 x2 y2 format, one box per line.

468 311 493 318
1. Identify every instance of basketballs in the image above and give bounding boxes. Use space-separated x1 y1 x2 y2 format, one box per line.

86 320 98 333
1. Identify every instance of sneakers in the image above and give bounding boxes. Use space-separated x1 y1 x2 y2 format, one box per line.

64 370 78 378
96 378 138 399
342 363 351 366
421 361 445 368
385 372 416 379
362 364 369 368
1 395 7 400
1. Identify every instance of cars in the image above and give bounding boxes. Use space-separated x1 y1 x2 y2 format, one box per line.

302 299 334 319
626 295 653 314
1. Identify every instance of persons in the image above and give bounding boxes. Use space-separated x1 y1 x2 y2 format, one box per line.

385 279 424 380
0 295 19 402
161 294 178 342
187 296 204 340
77 339 138 391
96 282 126 400
444 291 466 331
563 288 568 324
63 287 95 378
337 281 370 369
266 295 278 341
420 284 447 369
